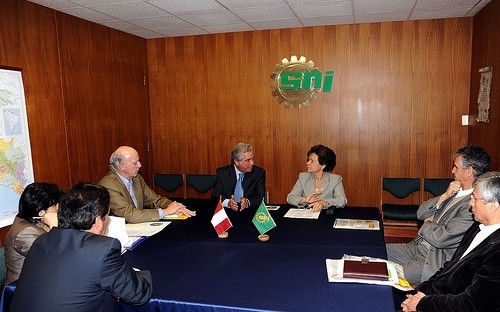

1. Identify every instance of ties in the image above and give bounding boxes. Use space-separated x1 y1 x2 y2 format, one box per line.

233 172 244 203
129 183 139 209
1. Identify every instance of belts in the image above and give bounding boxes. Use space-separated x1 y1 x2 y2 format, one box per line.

434 191 457 220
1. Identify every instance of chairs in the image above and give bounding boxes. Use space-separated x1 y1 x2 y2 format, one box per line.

152 173 216 199
380 177 455 227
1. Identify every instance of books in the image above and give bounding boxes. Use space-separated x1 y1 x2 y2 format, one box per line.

163 210 196 220
284 209 321 219
106 215 172 255
334 218 380 231
325 253 414 292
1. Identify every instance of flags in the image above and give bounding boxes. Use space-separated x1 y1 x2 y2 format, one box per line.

211 200 233 236
251 200 276 235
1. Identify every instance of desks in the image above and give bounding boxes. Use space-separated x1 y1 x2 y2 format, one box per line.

113 200 395 312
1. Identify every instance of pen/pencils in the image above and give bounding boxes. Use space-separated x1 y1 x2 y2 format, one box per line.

267 207 277 208
32 216 43 219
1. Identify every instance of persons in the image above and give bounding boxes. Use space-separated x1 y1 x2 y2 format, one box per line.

401 172 500 312
209 142 266 212
8 182 153 312
97 146 196 224
386 145 492 286
2 182 65 286
286 145 347 213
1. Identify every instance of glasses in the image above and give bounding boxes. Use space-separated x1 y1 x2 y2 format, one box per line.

471 192 484 202
